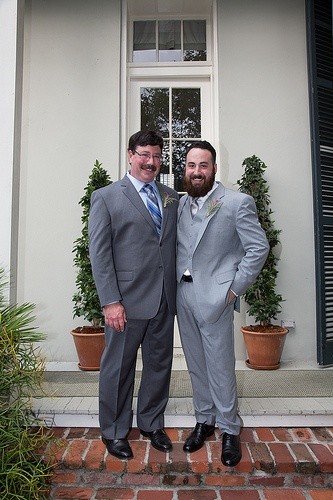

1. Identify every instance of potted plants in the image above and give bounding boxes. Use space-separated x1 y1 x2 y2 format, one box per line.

237 155 291 367
70 160 114 367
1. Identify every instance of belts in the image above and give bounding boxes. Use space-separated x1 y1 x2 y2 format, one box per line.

182 274 193 282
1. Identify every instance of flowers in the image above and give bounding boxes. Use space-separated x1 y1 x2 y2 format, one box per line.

207 197 221 216
161 192 177 208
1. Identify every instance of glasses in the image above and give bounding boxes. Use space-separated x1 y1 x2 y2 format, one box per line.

134 150 163 165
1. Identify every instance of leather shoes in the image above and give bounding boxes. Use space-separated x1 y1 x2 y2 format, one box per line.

139 428 173 453
183 423 215 453
102 435 134 460
221 432 242 467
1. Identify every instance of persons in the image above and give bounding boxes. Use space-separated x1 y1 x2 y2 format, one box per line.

88 130 179 458
176 141 270 467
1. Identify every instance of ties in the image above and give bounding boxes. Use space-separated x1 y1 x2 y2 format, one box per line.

190 197 199 219
142 184 162 239
